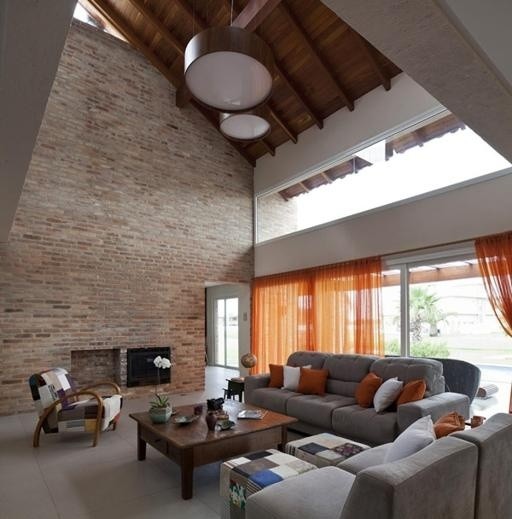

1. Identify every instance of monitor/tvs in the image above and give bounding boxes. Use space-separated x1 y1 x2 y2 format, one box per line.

126 347 172 387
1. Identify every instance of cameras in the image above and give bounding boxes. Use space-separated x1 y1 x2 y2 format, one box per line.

206 397 225 410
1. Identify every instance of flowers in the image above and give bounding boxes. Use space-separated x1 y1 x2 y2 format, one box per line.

149 349 173 408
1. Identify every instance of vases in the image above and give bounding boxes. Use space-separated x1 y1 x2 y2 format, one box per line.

149 404 172 423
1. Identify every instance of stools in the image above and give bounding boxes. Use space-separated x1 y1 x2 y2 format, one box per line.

284 432 372 468
218 449 318 519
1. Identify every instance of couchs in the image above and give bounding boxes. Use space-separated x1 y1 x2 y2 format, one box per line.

245 406 480 519
336 412 512 518
244 351 482 447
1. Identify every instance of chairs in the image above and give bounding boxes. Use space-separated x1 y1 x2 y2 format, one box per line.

28 366 123 447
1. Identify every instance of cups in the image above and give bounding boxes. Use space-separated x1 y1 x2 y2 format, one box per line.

194 404 202 415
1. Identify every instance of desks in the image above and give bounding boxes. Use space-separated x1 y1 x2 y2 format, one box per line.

128 398 300 500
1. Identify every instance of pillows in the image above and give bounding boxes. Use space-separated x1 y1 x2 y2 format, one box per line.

356 371 384 408
372 376 405 413
268 365 285 387
384 415 437 464
298 366 329 393
435 409 464 437
282 364 312 391
397 379 427 405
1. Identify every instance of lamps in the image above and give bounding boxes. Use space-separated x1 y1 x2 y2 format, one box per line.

215 109 271 143
184 1 276 113
